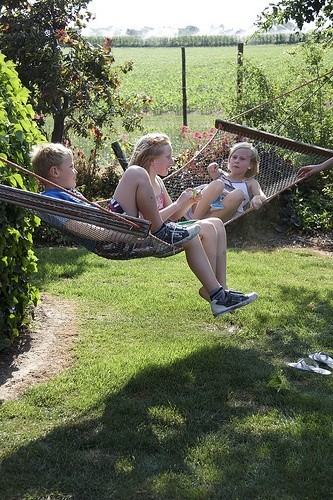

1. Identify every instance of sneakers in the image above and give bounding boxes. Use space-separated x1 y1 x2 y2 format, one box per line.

152 222 201 252
211 290 258 318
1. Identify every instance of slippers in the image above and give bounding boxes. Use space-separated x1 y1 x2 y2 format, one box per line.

288 358 331 375
308 351 333 369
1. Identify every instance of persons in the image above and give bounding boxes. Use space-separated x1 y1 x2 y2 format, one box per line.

31 143 201 260
182 143 267 222
110 133 258 317
